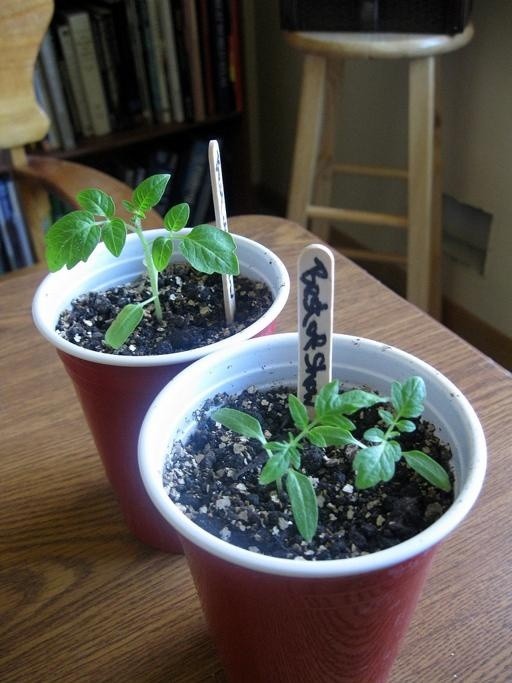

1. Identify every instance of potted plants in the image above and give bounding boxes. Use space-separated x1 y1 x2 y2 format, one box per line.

136 328 488 683
31 169 290 552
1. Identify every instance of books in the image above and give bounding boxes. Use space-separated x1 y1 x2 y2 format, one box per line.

124 141 212 228
1 179 34 275
26 0 245 151
40 186 75 233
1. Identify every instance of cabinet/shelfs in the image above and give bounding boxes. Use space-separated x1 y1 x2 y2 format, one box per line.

0 0 259 281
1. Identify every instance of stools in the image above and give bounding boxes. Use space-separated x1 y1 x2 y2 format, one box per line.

280 16 477 320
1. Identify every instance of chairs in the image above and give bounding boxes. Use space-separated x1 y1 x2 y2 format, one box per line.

0 1 165 253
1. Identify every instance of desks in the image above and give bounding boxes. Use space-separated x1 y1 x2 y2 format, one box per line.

0 214 512 683
